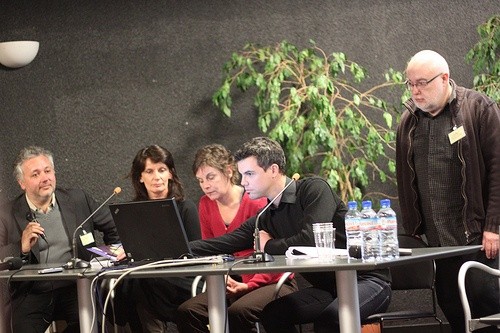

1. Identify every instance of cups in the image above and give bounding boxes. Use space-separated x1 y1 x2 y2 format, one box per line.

312 222 336 263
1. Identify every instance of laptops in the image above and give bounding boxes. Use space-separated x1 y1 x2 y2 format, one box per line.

109 197 235 261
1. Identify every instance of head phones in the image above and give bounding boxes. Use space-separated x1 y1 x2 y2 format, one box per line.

0 256 22 270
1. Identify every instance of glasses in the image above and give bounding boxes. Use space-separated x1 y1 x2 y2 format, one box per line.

404 73 442 88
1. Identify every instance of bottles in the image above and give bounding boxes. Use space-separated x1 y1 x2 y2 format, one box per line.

344 201 364 264
359 201 380 263
376 199 400 262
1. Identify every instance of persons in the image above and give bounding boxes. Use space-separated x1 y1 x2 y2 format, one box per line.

0 145 123 333
396 49 500 333
189 136 392 333
115 144 206 333
176 143 299 333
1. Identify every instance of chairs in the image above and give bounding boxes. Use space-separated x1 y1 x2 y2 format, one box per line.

52 233 500 333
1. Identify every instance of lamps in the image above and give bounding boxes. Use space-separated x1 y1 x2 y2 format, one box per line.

0 41 40 68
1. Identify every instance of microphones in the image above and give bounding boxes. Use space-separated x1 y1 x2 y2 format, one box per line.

26 211 50 264
61 187 121 268
244 173 300 263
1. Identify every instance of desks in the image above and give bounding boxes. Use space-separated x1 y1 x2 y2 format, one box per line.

0 243 484 333
